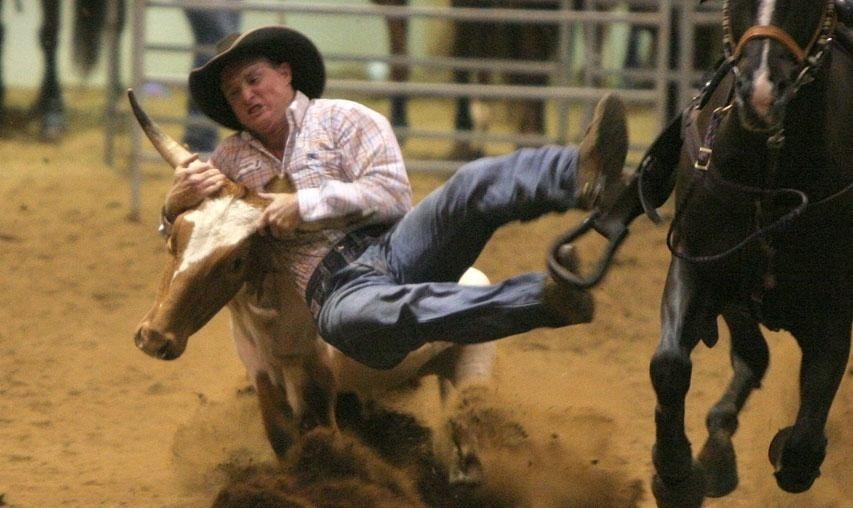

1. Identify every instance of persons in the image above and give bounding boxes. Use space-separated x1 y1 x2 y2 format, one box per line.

159 25 629 371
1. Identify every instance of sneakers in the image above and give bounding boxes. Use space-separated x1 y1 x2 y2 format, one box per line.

575 93 627 208
545 246 592 324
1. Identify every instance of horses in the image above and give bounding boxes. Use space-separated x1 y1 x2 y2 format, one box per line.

0 0 107 141
648 0 852 508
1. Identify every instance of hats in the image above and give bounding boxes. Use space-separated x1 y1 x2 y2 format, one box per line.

188 26 325 130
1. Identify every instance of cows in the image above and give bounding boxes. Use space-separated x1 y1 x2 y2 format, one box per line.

124 87 493 488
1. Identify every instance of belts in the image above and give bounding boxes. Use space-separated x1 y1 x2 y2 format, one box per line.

306 227 381 305
